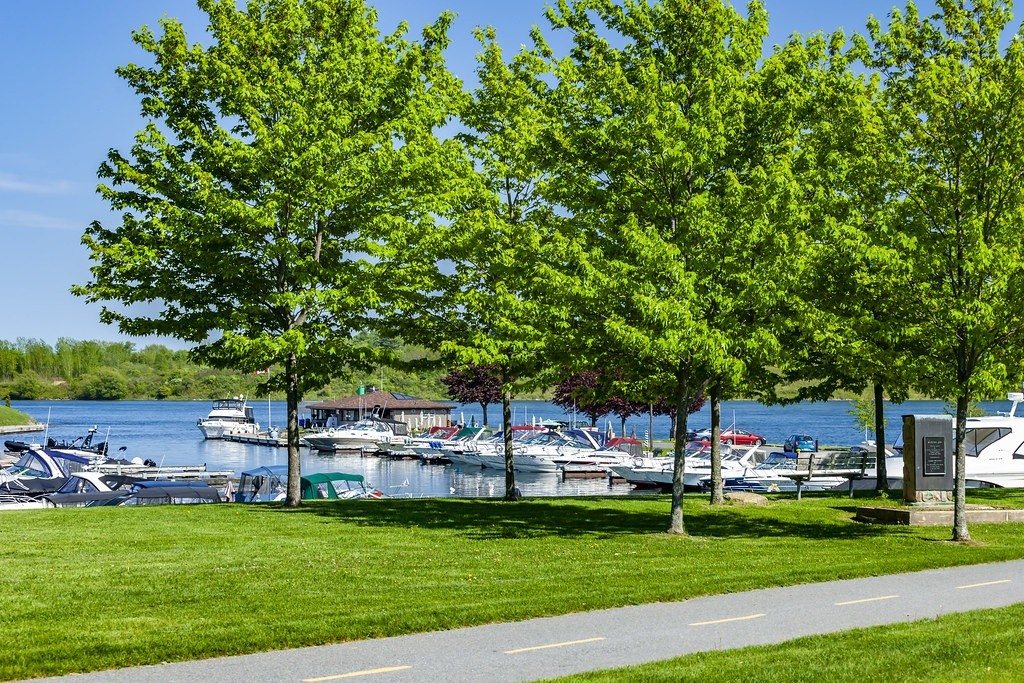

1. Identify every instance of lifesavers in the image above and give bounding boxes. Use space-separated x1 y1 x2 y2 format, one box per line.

634 458 644 467
329 431 334 436
495 444 503 453
219 419 222 423
521 446 528 453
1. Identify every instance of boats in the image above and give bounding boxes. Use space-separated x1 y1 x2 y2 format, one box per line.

0 392 1024 511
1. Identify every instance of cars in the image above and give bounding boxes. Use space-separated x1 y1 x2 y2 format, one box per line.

685 426 725 442
720 428 766 447
783 434 816 452
571 420 590 430
